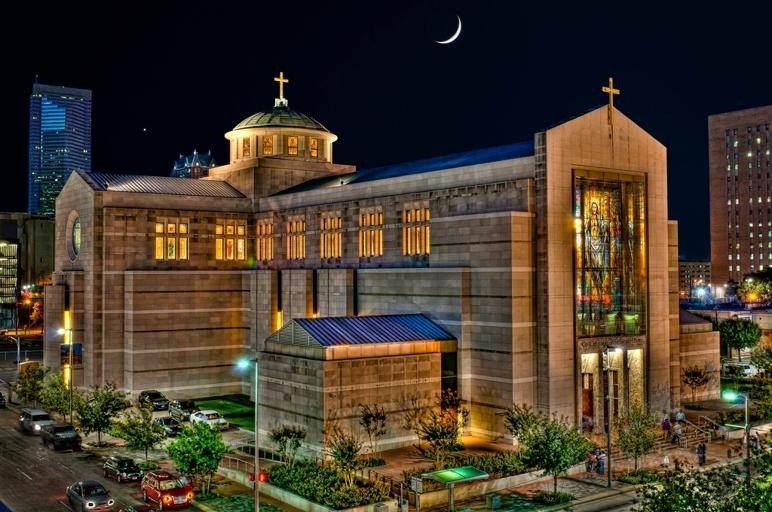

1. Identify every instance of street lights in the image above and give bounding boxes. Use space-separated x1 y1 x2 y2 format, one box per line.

236 357 259 511
59 326 73 429
722 392 750 475
604 341 627 489
8 335 20 371
745 279 753 303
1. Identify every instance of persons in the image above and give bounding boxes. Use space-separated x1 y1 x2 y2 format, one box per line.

583 406 764 477
584 201 611 303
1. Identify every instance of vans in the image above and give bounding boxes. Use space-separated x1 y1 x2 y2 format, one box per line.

719 362 750 376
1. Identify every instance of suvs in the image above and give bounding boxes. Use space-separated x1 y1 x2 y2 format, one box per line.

141 471 193 511
19 407 55 435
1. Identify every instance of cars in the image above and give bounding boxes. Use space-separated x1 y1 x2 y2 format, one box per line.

102 455 144 483
0 391 7 408
66 480 114 512
140 389 230 438
40 422 82 451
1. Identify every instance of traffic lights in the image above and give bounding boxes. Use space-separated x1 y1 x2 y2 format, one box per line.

248 471 269 482
